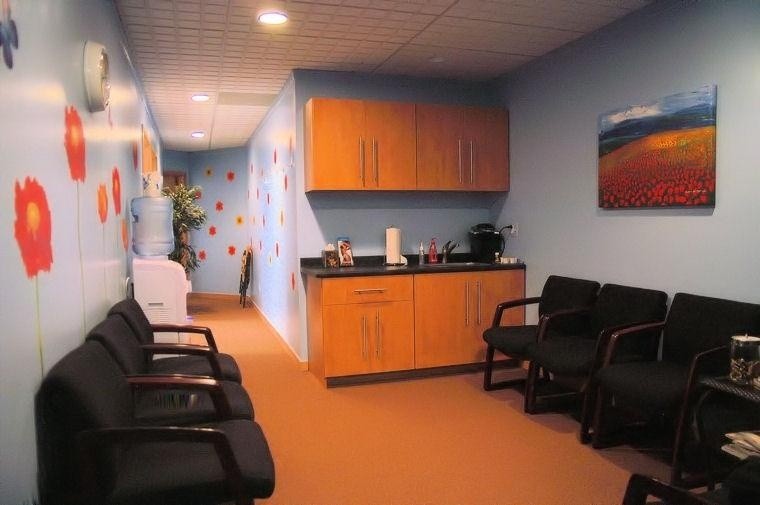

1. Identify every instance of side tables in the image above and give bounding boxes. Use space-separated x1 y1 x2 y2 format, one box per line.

668 377 756 484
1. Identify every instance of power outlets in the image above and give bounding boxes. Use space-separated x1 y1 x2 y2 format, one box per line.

509 223 519 234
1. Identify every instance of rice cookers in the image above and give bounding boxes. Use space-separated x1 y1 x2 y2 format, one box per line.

469 225 505 262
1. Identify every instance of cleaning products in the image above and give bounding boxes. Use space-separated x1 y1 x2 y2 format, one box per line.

428 238 437 264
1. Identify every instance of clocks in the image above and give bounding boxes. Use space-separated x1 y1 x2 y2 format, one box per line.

81 40 114 113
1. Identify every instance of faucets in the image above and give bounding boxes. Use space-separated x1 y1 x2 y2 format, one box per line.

443 239 462 262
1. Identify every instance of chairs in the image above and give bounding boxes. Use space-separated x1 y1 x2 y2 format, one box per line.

592 291 759 487
528 282 666 445
481 272 601 415
620 471 724 504
40 339 276 505
86 315 254 426
108 298 243 383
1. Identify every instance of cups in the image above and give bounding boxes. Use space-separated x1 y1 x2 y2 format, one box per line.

510 258 517 264
500 257 509 265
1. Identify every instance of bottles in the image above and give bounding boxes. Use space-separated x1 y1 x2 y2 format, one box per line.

495 252 499 262
428 239 439 263
419 241 426 265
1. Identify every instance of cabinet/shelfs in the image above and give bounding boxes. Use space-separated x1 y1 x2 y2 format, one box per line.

306 275 415 390
304 96 418 189
416 103 508 192
413 270 527 379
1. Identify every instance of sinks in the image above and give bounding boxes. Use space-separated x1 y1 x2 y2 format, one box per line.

425 263 491 268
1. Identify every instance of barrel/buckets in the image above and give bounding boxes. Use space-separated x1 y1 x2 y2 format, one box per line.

132 197 174 256
